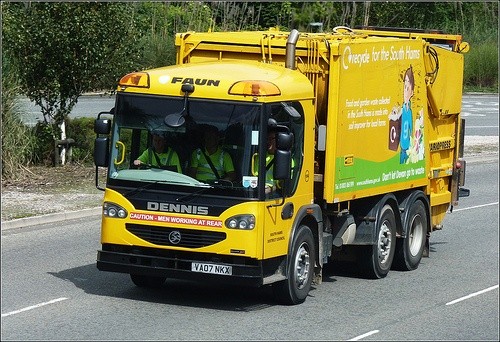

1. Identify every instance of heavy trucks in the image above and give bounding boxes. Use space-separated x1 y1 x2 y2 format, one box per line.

95 23 472 305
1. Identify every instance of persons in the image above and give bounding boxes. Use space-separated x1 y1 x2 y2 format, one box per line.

191 133 235 183
254 132 294 193
134 133 182 173
